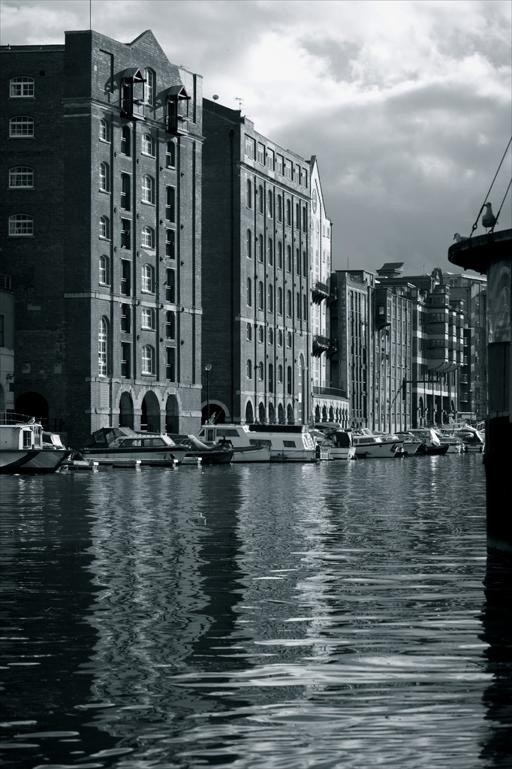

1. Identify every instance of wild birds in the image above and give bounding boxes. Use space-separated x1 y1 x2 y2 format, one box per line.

481 201 499 232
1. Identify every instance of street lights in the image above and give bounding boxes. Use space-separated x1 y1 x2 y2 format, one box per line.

204 362 212 424
